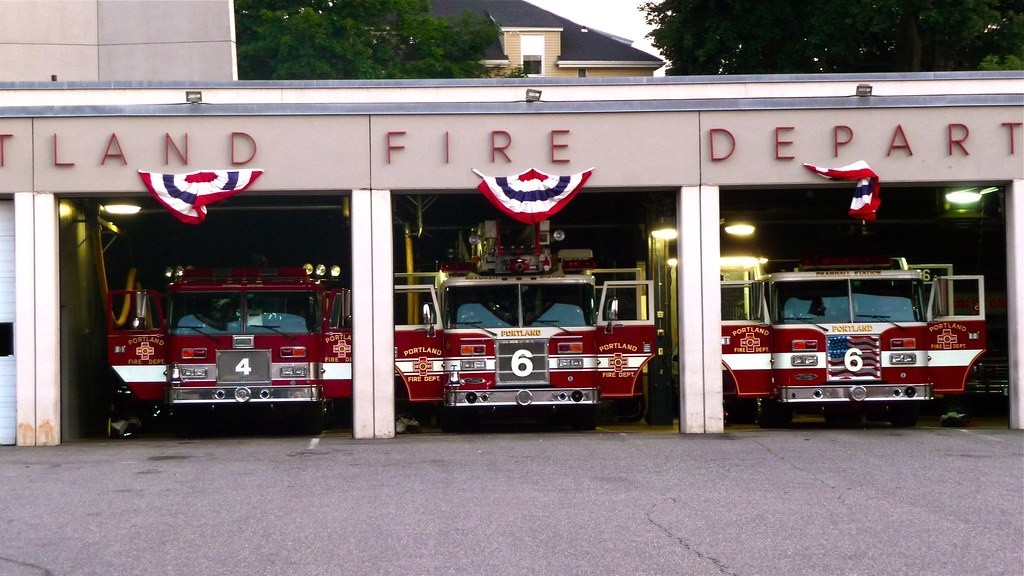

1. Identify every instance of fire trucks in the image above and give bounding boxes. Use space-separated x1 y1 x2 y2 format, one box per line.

393 217 656 433
103 263 353 440
719 253 987 430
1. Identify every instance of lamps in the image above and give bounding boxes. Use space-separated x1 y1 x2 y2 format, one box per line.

185 90 203 105
856 83 872 97
525 89 542 102
